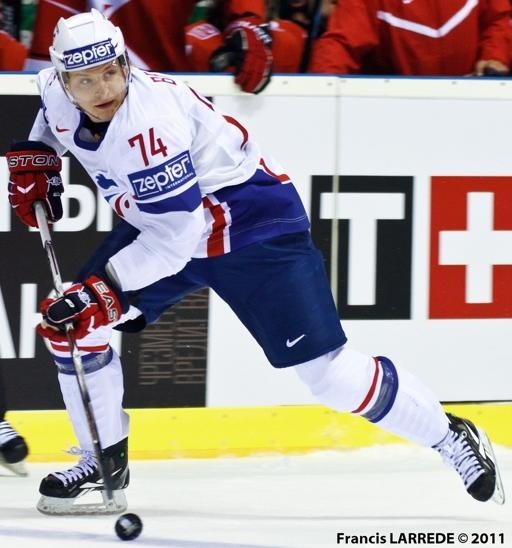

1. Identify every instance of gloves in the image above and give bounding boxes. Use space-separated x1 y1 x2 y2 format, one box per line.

6 141 64 228
36 272 129 343
210 12 274 94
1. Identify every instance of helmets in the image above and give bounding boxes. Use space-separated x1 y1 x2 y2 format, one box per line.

49 9 126 72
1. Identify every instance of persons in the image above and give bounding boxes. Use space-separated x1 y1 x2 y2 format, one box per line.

0 409 28 463
6 9 496 502
0 1 511 97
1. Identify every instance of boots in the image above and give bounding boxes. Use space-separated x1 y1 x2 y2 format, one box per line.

431 413 496 502
0 419 28 463
39 437 130 498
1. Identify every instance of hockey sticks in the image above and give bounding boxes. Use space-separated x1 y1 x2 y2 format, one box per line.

35 201 118 499
